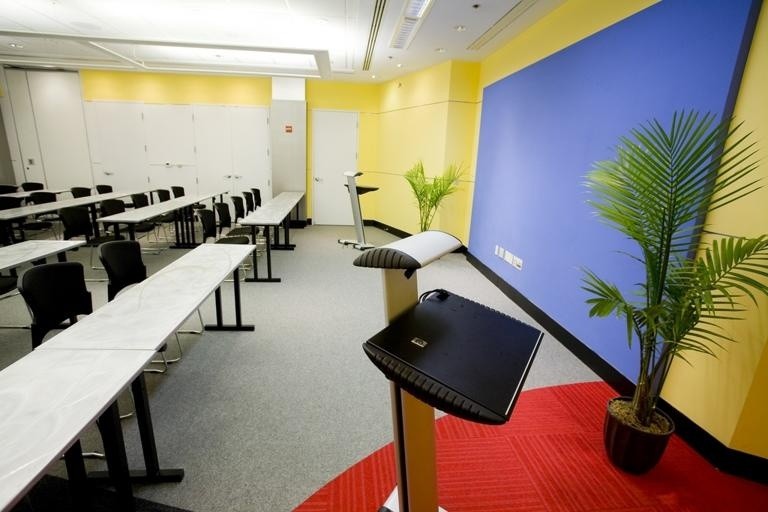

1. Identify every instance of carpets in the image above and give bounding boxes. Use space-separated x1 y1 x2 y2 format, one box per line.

289 381 768 512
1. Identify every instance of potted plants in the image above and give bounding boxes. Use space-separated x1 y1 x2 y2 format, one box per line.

573 107 768 476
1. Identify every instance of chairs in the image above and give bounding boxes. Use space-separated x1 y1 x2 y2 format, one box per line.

1 189 262 270
21 182 44 219
96 240 184 374
16 260 137 460
0 184 19 194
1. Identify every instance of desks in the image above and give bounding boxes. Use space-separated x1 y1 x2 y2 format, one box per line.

239 191 304 283
1 239 88 331
0 350 160 512
